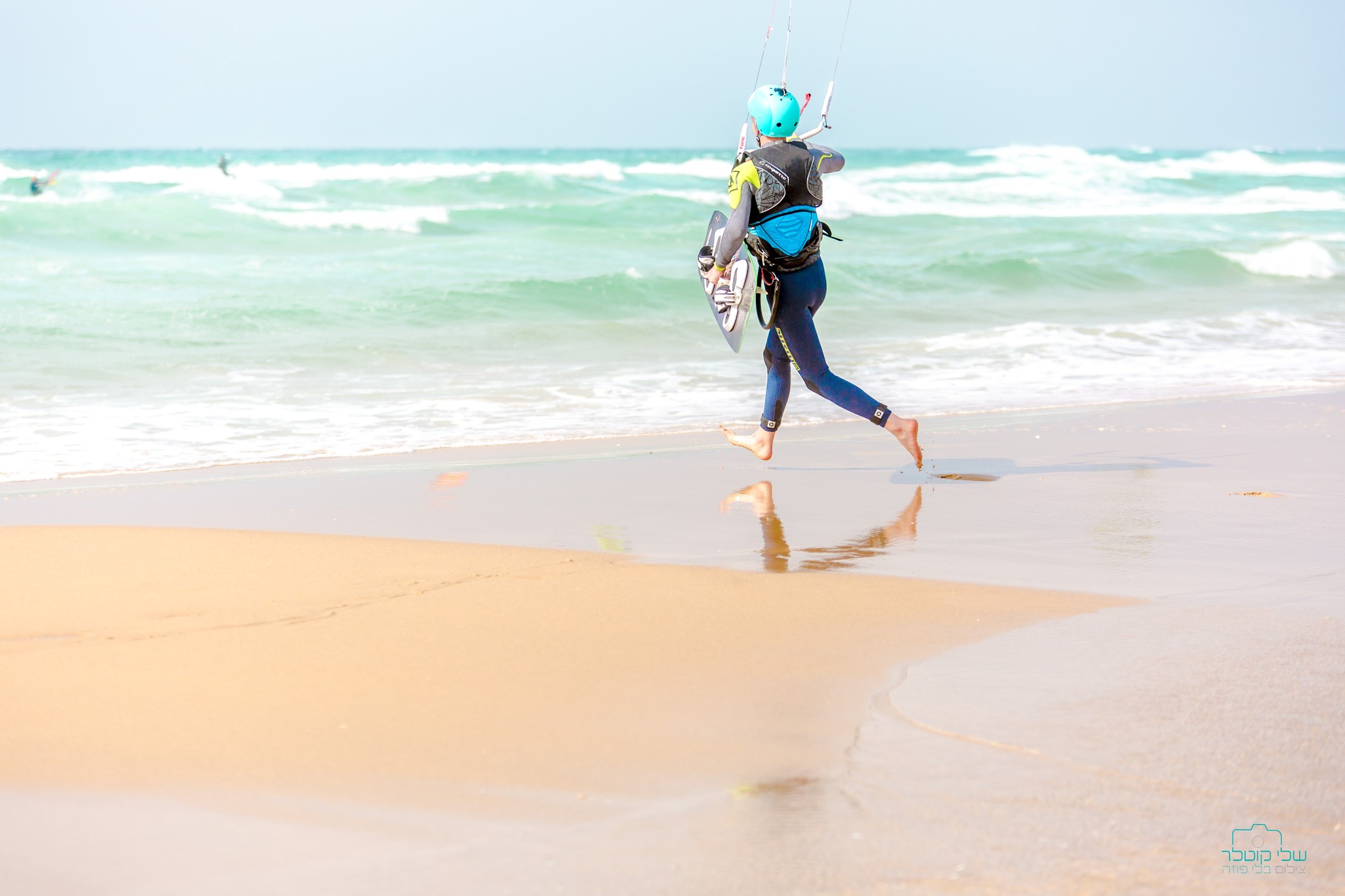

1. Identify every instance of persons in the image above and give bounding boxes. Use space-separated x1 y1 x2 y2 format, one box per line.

219 155 233 175
708 85 923 469
30 177 46 195
720 483 922 570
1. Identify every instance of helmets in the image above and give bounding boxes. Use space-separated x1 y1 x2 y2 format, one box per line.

747 85 800 137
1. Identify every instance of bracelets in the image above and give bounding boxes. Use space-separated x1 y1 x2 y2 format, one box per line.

715 261 725 270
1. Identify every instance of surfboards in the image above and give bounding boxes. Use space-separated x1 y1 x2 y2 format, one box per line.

697 209 758 354
